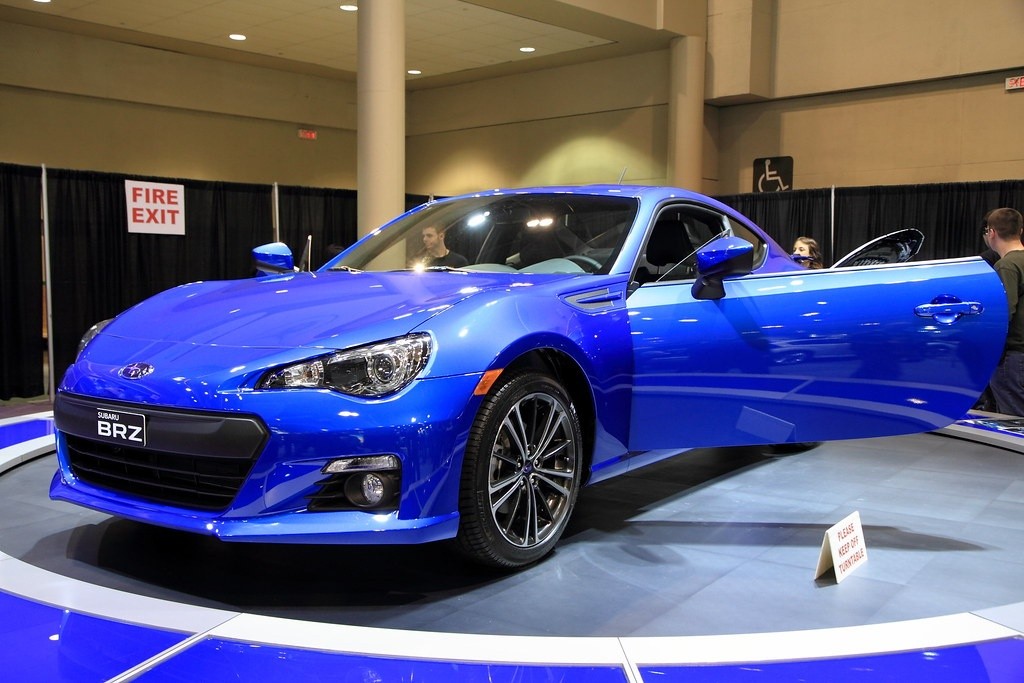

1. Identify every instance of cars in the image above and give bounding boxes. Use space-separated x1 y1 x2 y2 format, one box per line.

46 186 1008 569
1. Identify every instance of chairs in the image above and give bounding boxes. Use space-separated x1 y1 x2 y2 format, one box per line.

636 220 694 280
518 228 564 270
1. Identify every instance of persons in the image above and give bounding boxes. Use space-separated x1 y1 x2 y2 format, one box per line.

978 208 1024 417
793 237 823 269
417 220 469 267
